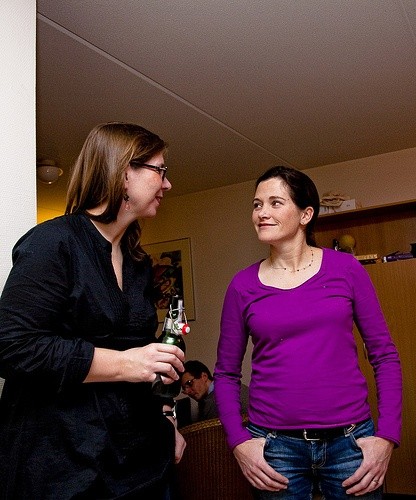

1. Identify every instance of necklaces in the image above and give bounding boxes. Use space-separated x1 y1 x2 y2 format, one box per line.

270 245 313 272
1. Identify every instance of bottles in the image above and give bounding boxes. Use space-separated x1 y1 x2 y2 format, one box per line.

150 296 185 398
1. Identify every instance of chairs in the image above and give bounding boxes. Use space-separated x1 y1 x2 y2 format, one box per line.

178 412 249 500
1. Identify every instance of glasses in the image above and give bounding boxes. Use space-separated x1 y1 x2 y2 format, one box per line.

129 161 167 181
182 379 194 391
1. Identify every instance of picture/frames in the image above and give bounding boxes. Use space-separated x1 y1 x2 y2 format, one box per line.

143 237 196 324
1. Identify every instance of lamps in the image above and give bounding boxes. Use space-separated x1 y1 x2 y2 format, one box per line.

36 153 64 184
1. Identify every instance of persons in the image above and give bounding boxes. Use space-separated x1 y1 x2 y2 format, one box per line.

0 122 187 500
181 360 250 422
213 166 404 500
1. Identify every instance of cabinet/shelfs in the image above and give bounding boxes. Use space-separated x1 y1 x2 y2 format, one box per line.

270 198 416 495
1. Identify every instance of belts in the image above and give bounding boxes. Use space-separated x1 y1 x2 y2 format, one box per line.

249 418 371 441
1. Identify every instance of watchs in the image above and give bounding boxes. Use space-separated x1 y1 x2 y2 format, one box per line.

162 410 177 420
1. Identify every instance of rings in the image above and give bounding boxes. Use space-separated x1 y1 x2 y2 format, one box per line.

152 374 165 394
372 479 379 484
